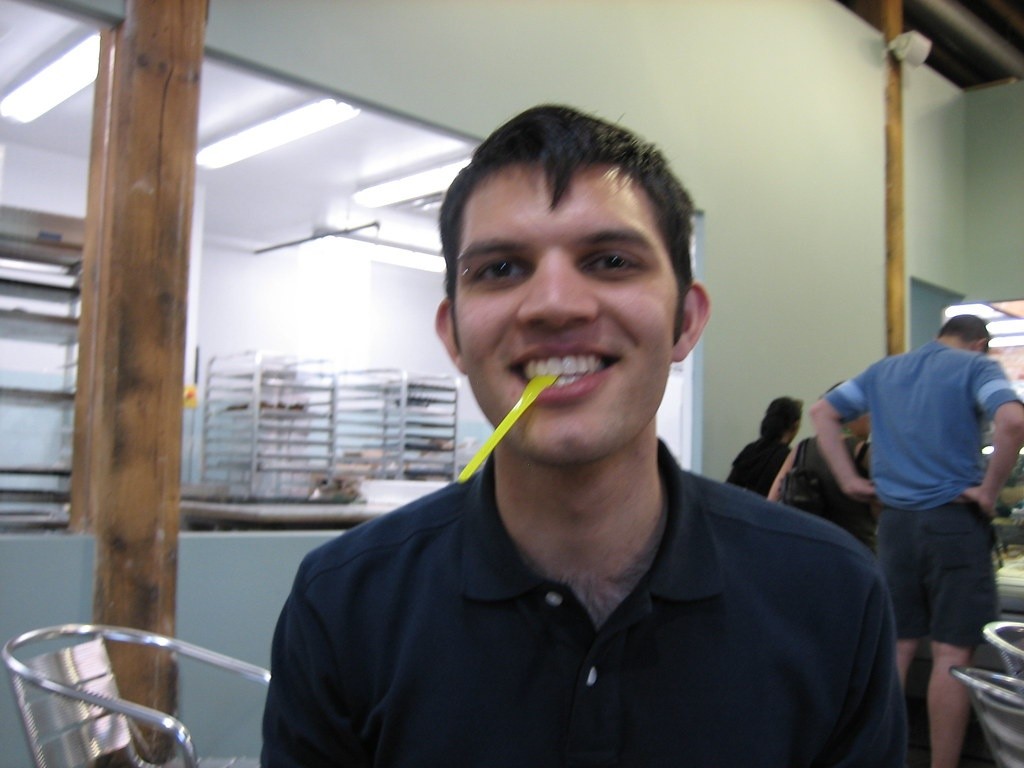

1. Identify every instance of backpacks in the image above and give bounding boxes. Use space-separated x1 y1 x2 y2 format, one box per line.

779 435 871 548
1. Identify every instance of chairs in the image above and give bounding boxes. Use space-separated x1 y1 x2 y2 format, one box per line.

2 624 272 768
983 620 1024 678
948 665 1024 768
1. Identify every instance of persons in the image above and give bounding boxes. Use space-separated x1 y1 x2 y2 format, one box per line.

259 106 910 768
727 316 1024 768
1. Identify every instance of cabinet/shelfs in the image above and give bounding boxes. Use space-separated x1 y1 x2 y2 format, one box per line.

206 354 458 506
0 234 84 532
